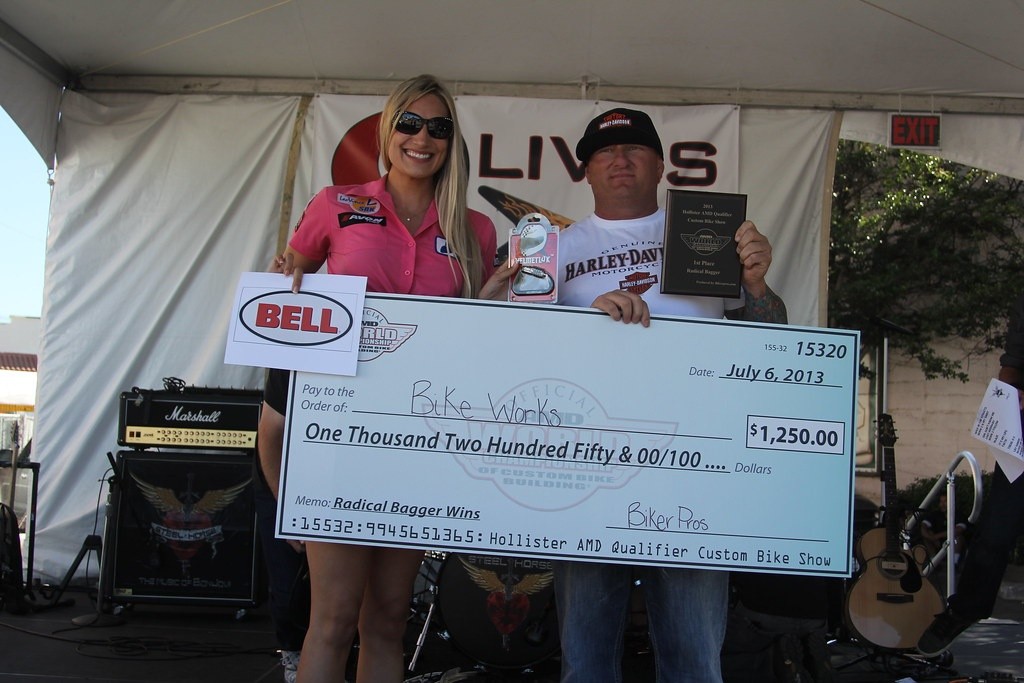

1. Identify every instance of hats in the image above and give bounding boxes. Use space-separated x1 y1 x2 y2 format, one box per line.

575 108 664 162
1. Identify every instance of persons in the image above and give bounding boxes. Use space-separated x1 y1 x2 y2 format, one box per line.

553 107 789 682
917 292 1024 658
271 74 520 683
258 369 306 553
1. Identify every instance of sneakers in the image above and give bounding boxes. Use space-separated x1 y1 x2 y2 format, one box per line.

918 604 981 658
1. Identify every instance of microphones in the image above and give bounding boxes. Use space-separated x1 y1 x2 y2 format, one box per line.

523 608 551 647
869 313 914 335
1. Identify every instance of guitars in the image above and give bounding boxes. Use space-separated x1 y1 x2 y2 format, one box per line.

6 419 21 509
848 414 946 648
436 552 559 671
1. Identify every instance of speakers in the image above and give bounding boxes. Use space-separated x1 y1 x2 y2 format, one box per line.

99 450 266 608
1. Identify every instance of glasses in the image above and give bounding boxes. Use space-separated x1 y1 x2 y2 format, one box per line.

391 110 454 139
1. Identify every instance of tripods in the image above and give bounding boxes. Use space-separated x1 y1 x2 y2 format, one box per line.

833 325 958 676
